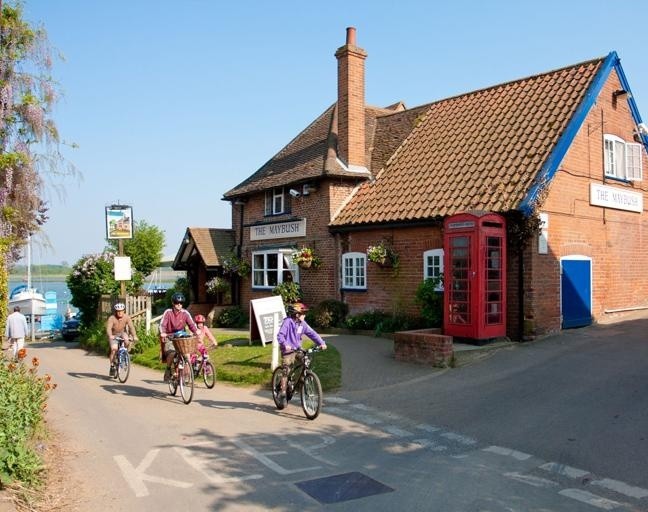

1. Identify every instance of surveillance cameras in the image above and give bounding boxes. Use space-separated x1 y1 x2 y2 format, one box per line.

290 189 300 198
638 123 648 135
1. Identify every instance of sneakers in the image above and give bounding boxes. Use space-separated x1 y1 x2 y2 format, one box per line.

164 369 172 382
278 393 288 409
109 367 115 376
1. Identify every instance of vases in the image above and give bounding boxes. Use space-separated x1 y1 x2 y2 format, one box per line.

298 260 312 270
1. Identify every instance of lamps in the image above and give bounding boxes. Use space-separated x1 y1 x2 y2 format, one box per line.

612 88 631 101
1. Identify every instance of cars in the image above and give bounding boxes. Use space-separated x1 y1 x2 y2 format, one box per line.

61 310 89 342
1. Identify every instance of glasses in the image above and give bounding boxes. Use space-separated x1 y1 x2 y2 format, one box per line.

173 301 184 305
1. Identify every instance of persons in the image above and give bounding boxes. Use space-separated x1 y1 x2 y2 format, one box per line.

105 301 141 380
190 314 218 369
3 304 29 357
277 301 331 401
157 289 200 385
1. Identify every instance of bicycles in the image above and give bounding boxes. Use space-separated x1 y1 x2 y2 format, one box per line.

111 338 135 383
271 345 323 422
192 345 218 389
162 334 196 404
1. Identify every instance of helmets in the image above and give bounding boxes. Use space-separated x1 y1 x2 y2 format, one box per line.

194 314 205 323
289 302 310 315
114 303 125 310
171 292 186 304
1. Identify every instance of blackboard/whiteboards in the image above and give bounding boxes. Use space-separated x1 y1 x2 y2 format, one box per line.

251 296 288 342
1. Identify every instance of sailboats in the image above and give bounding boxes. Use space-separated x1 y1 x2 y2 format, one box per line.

8 232 48 318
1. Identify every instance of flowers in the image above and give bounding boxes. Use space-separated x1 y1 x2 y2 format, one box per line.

222 257 250 276
204 275 228 297
292 247 314 264
365 243 385 263
